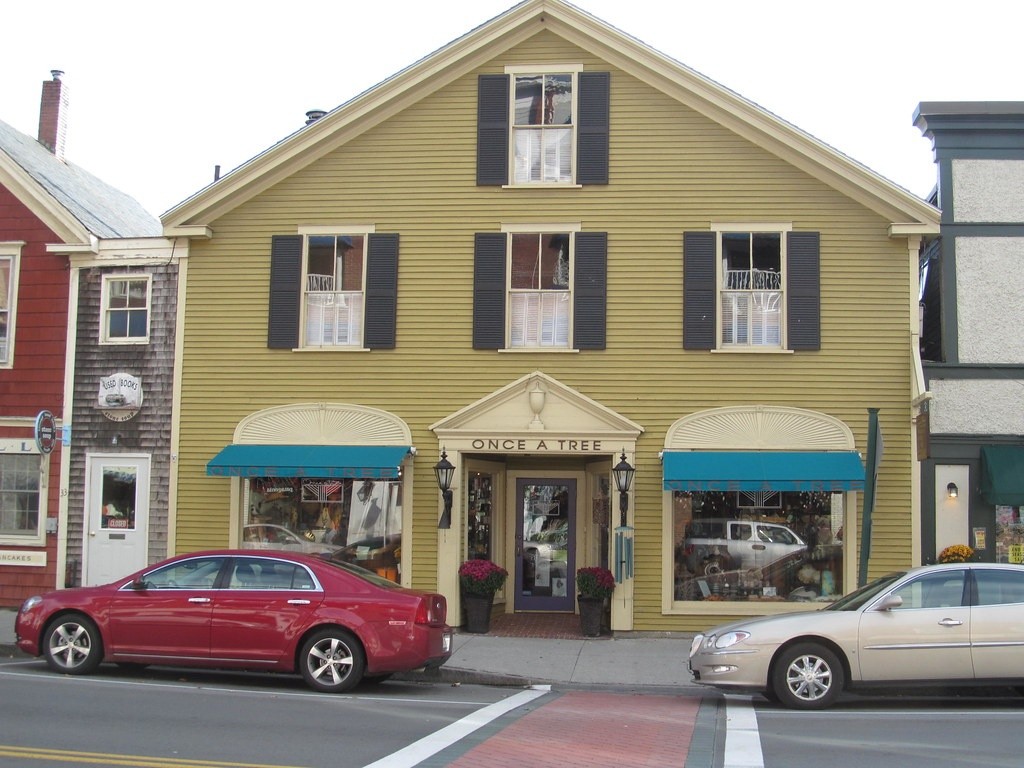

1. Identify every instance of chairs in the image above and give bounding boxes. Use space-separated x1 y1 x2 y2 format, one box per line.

235 566 278 587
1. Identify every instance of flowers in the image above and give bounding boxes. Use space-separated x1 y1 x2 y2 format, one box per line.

577 566 617 598
459 560 509 593
939 544 974 563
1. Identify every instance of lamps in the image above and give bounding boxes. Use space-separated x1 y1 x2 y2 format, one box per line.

947 483 959 497
431 446 456 529
612 445 635 528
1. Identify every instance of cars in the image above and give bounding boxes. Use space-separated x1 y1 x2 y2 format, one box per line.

320 534 402 571
244 523 345 556
682 517 808 573
16 545 452 688
684 557 1024 709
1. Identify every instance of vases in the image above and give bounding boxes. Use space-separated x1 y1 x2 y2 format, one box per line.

466 592 495 633
577 594 605 638
